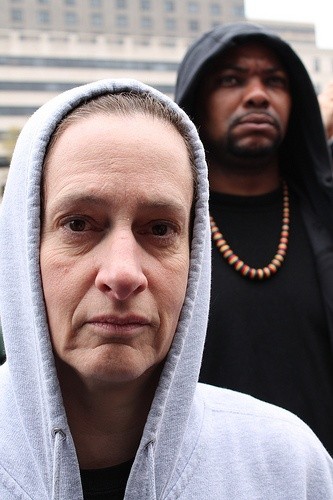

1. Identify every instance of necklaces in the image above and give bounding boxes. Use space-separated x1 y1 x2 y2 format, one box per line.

209 180 289 279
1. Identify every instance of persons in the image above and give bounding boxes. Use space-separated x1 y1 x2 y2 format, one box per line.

0 22 333 500
